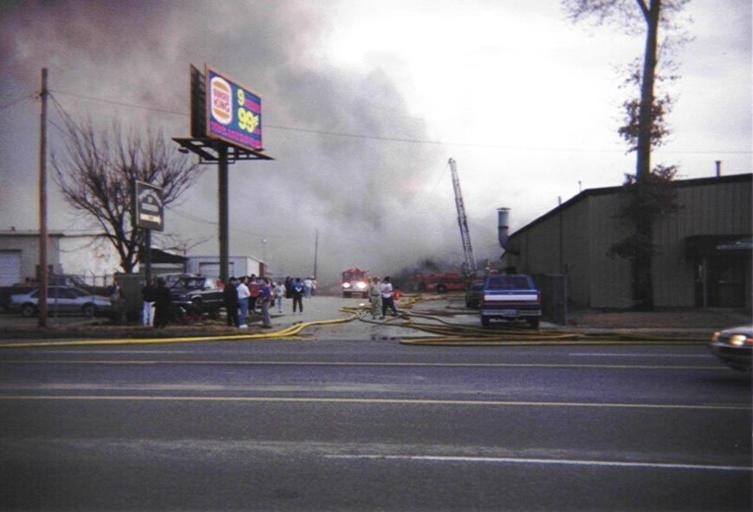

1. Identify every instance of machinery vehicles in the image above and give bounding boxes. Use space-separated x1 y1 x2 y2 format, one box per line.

415 158 477 294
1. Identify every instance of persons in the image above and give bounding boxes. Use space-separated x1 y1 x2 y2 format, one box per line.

23 277 32 287
381 275 397 319
104 278 126 305
141 275 172 329
367 275 373 303
222 272 317 329
368 275 383 319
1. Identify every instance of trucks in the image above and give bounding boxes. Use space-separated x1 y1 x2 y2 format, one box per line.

341 267 371 299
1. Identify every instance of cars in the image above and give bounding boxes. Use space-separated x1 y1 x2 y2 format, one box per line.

10 285 112 317
710 324 753 372
161 274 276 315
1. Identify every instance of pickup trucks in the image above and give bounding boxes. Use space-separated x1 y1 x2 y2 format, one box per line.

465 273 542 330
